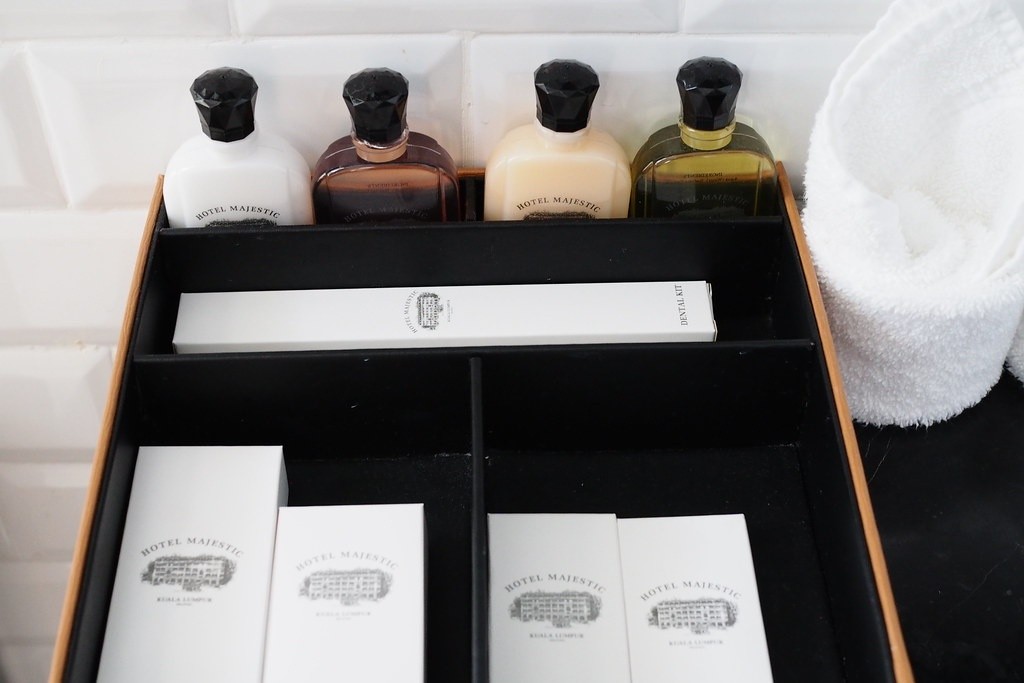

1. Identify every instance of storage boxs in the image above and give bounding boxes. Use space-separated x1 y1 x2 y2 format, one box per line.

47 161 915 683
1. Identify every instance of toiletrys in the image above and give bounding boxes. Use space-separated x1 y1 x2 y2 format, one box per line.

91 54 782 683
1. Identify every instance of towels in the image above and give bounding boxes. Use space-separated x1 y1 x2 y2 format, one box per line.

800 1 1024 431
